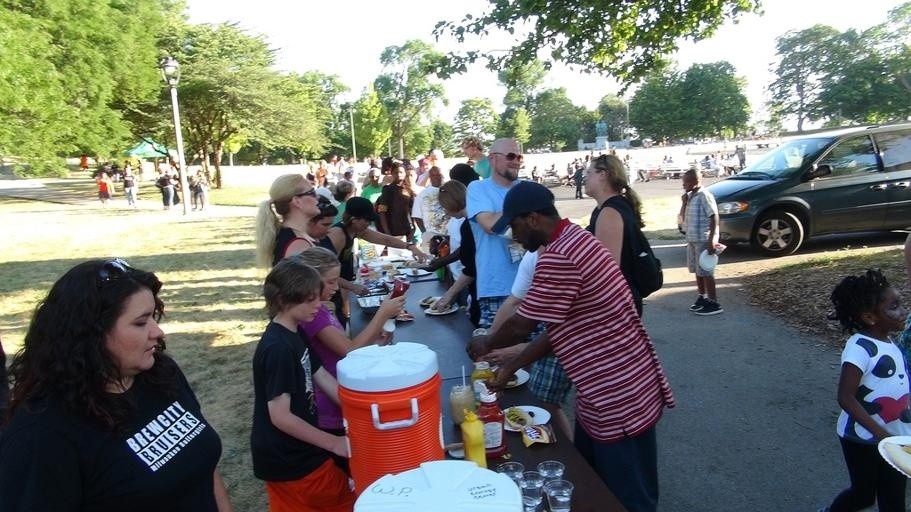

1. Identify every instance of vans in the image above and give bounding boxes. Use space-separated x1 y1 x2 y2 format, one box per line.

677 125 911 253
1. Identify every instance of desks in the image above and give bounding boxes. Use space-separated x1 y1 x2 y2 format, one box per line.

432 377 626 511
353 246 440 284
347 280 491 383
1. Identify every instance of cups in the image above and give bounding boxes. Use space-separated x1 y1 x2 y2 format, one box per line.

497 460 574 512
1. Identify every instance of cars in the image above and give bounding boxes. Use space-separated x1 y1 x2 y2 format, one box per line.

513 168 562 190
636 157 752 181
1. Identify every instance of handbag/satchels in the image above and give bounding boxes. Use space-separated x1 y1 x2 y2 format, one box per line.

625 239 663 296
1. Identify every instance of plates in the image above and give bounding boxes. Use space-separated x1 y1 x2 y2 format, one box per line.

356 255 428 315
491 364 531 389
699 250 720 272
499 405 552 432
420 295 460 316
878 433 911 481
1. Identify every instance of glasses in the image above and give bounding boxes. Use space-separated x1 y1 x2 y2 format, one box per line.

96 257 130 285
493 152 524 162
299 187 316 197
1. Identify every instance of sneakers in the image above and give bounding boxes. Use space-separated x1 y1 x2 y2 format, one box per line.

689 294 723 315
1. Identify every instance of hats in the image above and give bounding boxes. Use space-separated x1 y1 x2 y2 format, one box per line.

382 156 403 168
492 180 554 233
346 197 380 220
458 138 481 149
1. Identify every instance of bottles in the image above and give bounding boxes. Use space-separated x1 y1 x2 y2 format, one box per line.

463 411 488 468
475 389 510 459
474 380 489 409
471 361 495 389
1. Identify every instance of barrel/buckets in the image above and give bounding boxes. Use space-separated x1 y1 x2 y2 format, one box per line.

352 459 525 512
336 340 447 499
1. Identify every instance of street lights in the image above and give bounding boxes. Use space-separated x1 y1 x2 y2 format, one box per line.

616 96 632 142
347 102 358 159
160 52 196 219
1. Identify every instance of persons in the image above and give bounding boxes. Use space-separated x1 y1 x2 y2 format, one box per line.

705 144 746 175
251 147 446 512
80 154 208 211
0 256 233 512
897 232 911 375
678 169 724 315
432 137 676 512
816 271 911 512
